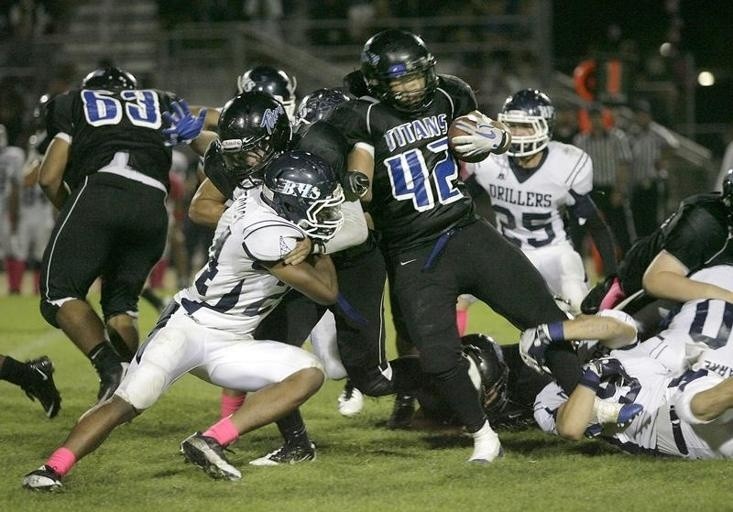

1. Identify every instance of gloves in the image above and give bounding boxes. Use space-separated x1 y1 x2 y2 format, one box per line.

592 359 632 382
452 115 509 158
517 324 553 375
162 101 207 138
347 171 369 198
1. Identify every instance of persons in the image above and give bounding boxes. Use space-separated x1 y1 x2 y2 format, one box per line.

2 15 733 498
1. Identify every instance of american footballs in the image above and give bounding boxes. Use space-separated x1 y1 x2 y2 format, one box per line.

448 116 490 163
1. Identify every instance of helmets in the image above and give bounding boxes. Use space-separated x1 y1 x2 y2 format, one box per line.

293 88 351 127
81 67 138 92
458 333 510 413
498 88 556 158
216 91 293 189
262 151 345 240
235 66 298 122
361 29 439 113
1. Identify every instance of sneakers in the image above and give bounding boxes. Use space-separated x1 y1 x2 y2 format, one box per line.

180 431 242 480
21 356 61 419
248 440 317 466
386 394 415 430
466 433 504 464
77 362 130 425
22 465 64 494
337 380 364 417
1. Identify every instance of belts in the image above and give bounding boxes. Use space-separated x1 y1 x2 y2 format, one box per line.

670 405 688 455
